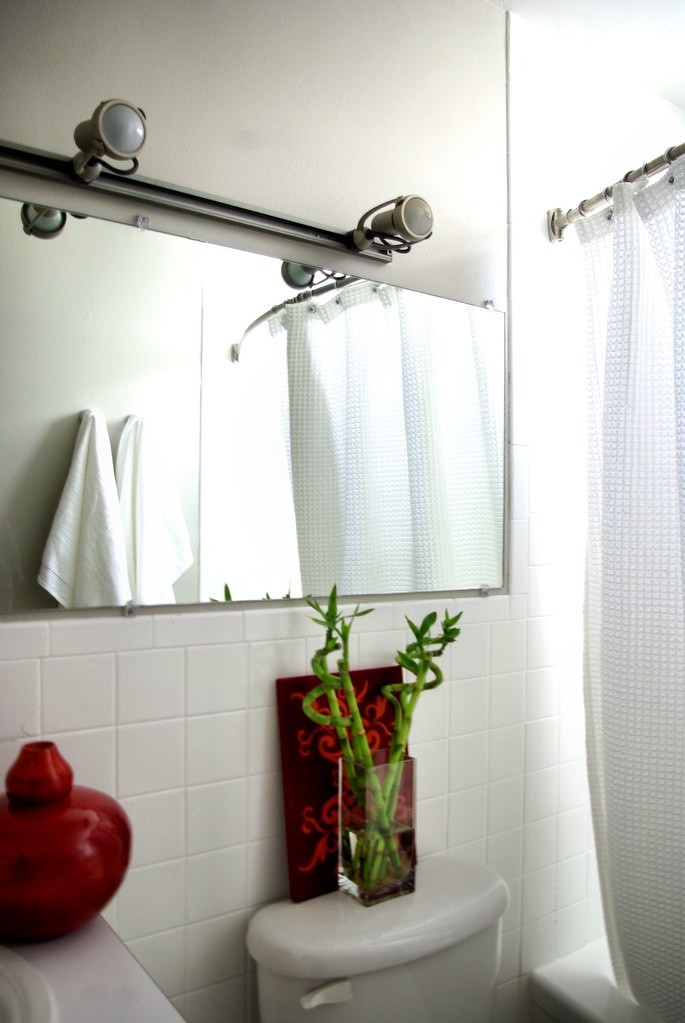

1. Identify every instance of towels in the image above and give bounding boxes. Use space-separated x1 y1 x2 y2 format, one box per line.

115 412 194 605
36 407 133 608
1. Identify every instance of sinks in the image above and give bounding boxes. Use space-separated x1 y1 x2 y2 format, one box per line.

0 944 59 1023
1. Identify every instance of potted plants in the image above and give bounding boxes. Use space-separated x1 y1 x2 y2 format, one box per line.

294 581 467 908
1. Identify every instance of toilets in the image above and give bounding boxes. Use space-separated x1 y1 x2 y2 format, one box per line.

249 854 510 1023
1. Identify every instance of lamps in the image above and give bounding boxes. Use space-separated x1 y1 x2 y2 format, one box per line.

20 201 68 239
70 98 147 182
340 194 435 256
281 261 347 290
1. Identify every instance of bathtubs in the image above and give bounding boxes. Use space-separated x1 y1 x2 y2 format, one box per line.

531 937 667 1023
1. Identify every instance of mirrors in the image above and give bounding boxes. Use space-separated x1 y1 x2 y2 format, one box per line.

0 195 506 619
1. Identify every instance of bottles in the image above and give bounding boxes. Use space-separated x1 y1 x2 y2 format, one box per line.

0 739 132 947
